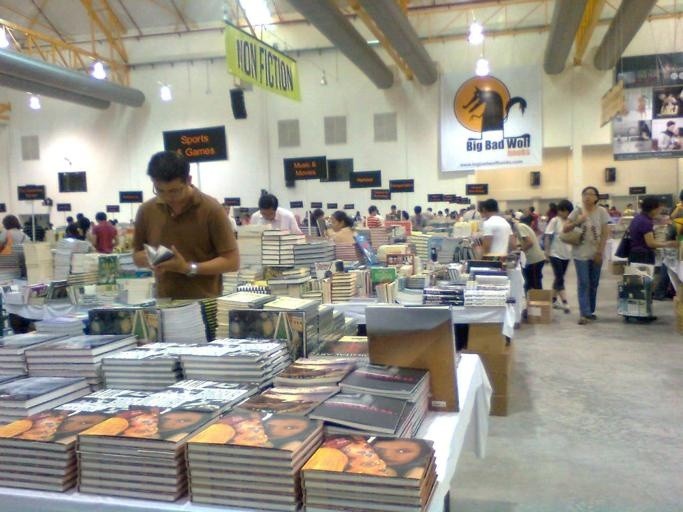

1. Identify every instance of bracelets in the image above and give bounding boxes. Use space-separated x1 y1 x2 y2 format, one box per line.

596 252 602 257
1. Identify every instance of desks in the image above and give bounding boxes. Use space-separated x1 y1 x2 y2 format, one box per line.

2 272 512 416
2 349 492 512
604 230 683 333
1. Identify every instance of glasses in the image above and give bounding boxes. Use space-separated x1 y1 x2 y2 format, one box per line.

152 182 185 198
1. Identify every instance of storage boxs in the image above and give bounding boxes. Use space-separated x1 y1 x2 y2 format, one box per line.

522 288 554 322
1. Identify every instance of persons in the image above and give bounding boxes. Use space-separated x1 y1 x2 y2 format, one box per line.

474 198 510 255
130 151 241 299
2 212 136 276
221 201 236 225
478 199 489 220
521 250 541 299
304 205 469 243
562 186 612 325
512 220 546 320
249 194 303 235
651 189 682 300
617 80 682 151
614 196 679 284
543 200 575 312
508 200 635 240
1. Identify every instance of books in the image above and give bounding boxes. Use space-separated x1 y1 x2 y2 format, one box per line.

1 239 153 306
1 285 463 511
143 244 174 266
221 217 511 307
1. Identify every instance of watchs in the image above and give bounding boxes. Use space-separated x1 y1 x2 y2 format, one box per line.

187 262 197 278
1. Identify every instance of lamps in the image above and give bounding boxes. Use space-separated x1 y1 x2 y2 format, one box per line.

467 10 487 47
28 92 43 112
154 79 176 104
475 42 492 79
88 57 109 82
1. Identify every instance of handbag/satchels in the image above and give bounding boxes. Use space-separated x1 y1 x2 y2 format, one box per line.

1 228 13 256
614 230 631 258
558 205 584 246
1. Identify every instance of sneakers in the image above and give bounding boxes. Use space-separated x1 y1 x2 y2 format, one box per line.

552 298 600 325
